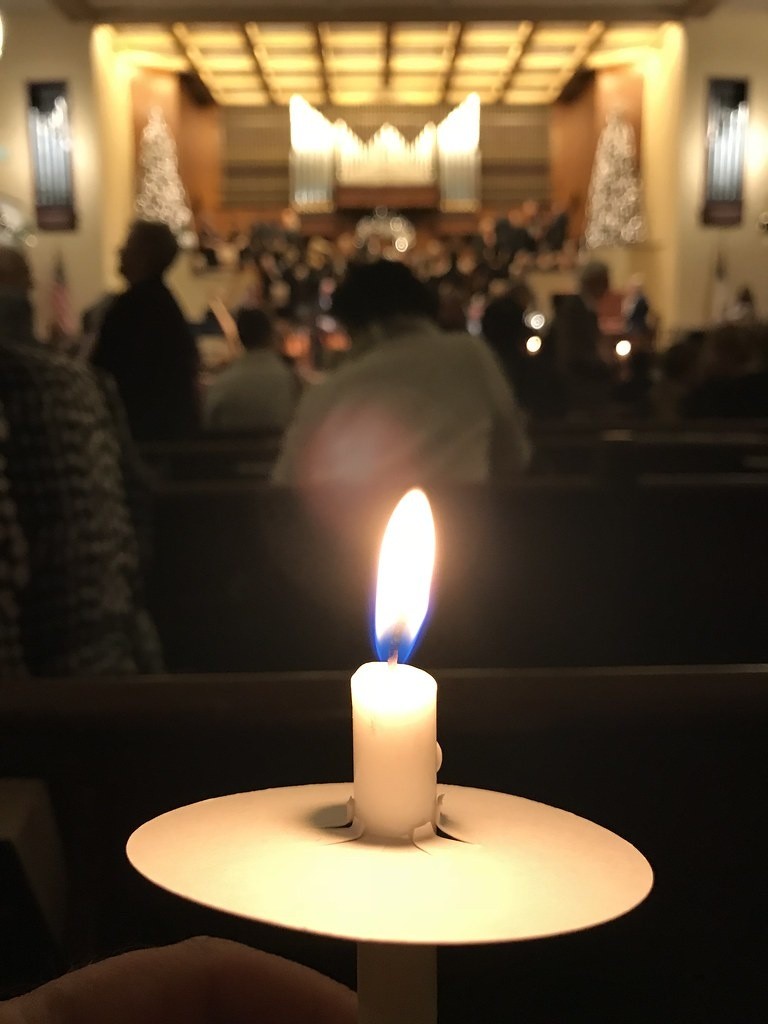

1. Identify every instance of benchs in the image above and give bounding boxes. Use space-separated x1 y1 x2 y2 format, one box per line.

0 404 768 934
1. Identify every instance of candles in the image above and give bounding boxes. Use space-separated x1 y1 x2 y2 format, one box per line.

351 487 436 835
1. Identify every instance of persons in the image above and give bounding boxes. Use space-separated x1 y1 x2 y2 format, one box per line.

0 208 768 494
0 342 165 677
0 935 360 1024
